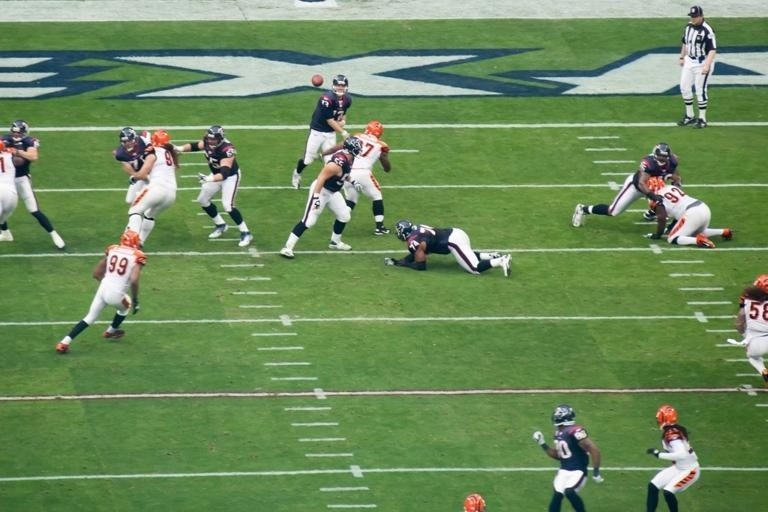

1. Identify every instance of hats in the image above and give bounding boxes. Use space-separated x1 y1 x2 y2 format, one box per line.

688 5 704 17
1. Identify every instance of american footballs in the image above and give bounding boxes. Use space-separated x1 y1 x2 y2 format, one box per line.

312 75 322 87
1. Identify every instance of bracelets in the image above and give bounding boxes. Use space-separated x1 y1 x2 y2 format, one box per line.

594 467 599 477
540 443 550 451
679 57 685 60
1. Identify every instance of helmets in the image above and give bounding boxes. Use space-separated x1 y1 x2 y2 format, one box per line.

343 136 363 159
120 230 142 249
10 120 30 141
394 220 414 241
207 125 224 149
150 129 169 146
463 493 485 512
645 175 666 191
752 274 768 293
119 127 138 152
364 120 384 139
551 404 576 427
656 404 678 430
332 75 348 97
652 143 672 166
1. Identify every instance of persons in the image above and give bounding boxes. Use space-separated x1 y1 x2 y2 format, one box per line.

383 219 511 277
732 273 768 382
569 143 683 229
0 141 19 223
172 125 254 247
55 230 148 354
643 404 702 512
0 119 67 249
678 5 716 131
461 491 489 512
532 403 606 512
646 175 734 247
113 127 179 247
292 75 352 189
279 120 391 259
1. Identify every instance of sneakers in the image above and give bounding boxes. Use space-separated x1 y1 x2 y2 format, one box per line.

328 238 352 250
500 254 512 277
101 329 125 338
642 209 656 221
572 204 585 227
292 168 302 186
692 118 706 129
696 233 715 248
279 248 294 257
49 230 66 248
0 229 14 242
238 231 253 246
56 342 69 351
373 222 390 235
489 252 500 258
208 224 228 238
720 228 732 240
678 115 696 126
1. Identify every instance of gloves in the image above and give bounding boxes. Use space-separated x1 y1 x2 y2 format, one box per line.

591 475 604 483
384 258 395 266
353 180 363 190
128 175 137 185
647 448 659 458
198 173 213 184
646 192 664 202
532 430 546 446
